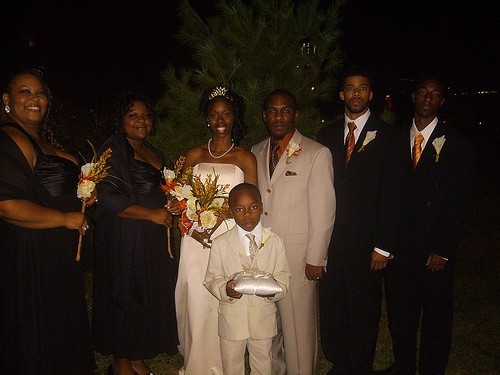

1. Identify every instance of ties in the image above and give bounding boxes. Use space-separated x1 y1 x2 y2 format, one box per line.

344 122 357 167
270 143 278 179
412 133 424 170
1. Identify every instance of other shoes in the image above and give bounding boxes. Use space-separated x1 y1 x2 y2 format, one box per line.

108 362 153 375
379 362 417 375
326 367 345 375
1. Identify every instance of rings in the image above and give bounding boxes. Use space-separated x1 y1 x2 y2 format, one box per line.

81 225 86 231
85 224 90 229
94 196 99 203
317 277 320 279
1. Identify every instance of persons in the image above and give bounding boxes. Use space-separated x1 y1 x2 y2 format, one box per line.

175 86 259 375
0 65 99 375
376 74 475 375
88 89 182 375
314 69 410 375
251 90 336 375
203 183 290 375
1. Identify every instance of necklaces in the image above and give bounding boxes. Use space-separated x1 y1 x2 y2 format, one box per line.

208 137 236 158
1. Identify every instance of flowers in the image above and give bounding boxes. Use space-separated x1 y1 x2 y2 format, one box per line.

432 136 446 162
259 226 274 250
75 137 132 261
357 130 378 152
285 141 301 164
160 155 230 257
